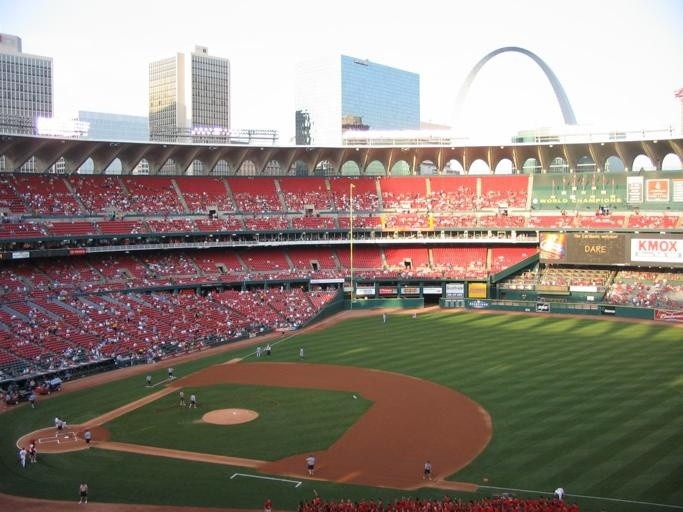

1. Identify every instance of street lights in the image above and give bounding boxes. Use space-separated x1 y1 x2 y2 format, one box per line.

349 183 355 301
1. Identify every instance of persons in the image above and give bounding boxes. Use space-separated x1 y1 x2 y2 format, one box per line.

306 454 316 476
553 486 565 500
382 313 387 323
346 189 683 310
78 480 89 504
421 459 434 481
15 415 73 468
0 171 346 410
83 430 91 446
412 311 417 322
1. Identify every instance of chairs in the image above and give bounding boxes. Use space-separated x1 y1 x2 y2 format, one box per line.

0 170 346 382
327 171 538 283
505 266 683 313
527 211 683 236
